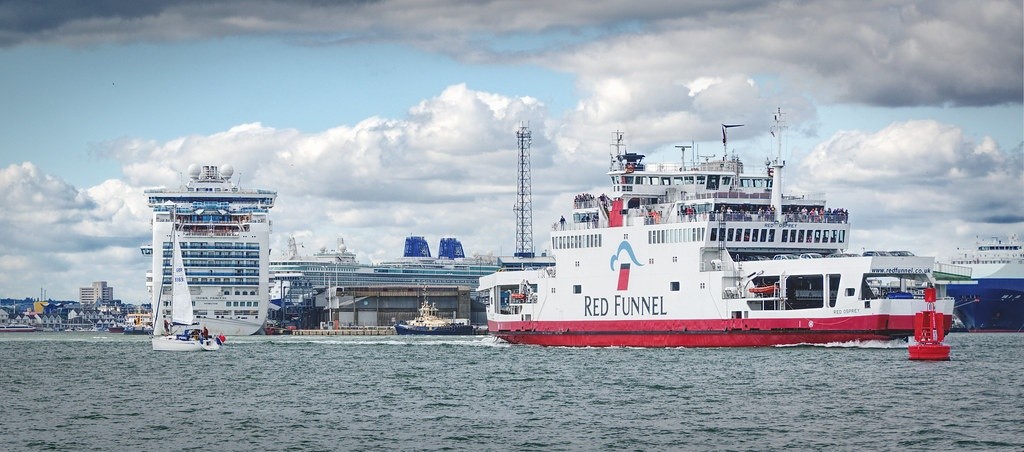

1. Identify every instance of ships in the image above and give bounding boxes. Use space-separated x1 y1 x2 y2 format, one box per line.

479 108 956 348
140 163 278 338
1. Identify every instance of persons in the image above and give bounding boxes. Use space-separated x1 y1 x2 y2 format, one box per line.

649 210 661 223
604 206 609 218
574 193 605 208
745 234 748 240
559 216 565 231
716 205 849 224
164 320 171 336
687 207 697 222
581 213 599 229
807 235 812 242
194 327 209 346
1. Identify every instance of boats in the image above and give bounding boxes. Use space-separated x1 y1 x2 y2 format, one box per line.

108 326 125 334
392 284 478 337
150 319 225 352
0 324 37 332
929 233 1024 333
